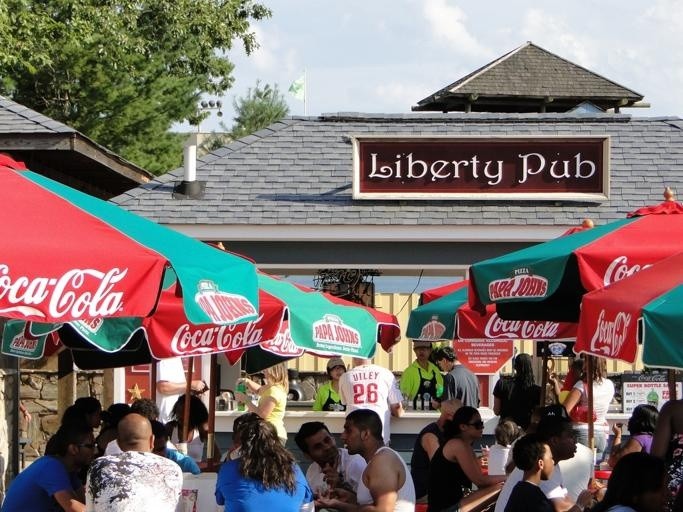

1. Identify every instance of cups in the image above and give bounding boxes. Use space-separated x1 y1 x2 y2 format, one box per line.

216 400 231 411
233 385 246 411
333 401 340 412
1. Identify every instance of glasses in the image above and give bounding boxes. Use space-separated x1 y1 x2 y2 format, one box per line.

462 419 487 429
76 442 94 450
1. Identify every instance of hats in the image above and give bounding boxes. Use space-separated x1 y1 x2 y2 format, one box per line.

325 357 346 369
412 341 432 349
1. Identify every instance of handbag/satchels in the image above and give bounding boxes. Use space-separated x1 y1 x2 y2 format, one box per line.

565 398 597 423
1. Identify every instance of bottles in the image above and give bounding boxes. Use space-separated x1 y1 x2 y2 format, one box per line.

647 388 659 410
423 393 429 411
415 393 423 411
401 390 414 411
